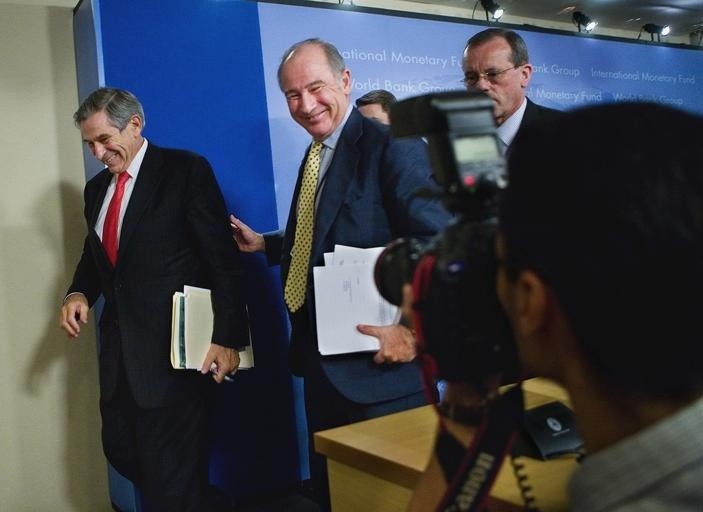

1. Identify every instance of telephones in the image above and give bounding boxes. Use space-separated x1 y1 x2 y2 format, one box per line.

510 399 586 460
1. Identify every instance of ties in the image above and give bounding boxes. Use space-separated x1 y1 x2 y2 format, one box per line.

284 141 323 313
102 171 129 269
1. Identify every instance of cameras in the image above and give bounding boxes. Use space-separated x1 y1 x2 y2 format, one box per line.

373 91 514 384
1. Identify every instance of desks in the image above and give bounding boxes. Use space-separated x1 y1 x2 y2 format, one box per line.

313 370 581 512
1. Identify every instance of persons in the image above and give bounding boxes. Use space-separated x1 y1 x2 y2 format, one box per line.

403 102 703 512
464 31 566 155
59 87 245 512
355 90 395 126
229 37 449 512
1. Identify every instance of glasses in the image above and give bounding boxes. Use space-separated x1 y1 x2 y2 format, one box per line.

460 66 515 85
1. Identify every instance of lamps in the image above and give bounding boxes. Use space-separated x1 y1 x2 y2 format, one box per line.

572 11 598 34
638 23 671 43
471 0 505 24
